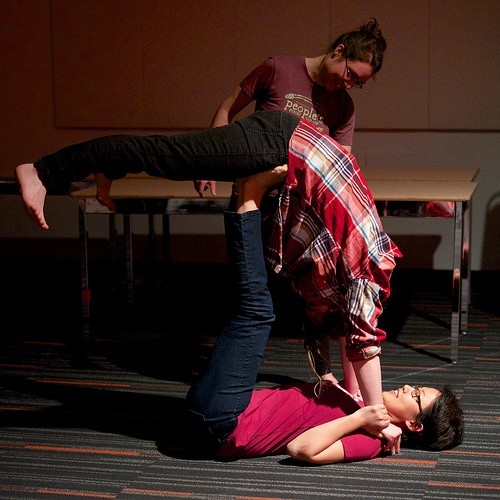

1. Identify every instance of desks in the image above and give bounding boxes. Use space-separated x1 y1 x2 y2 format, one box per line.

69 168 478 366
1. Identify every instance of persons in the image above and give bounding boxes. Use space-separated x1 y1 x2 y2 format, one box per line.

19 109 404 457
192 17 387 385
183 165 464 465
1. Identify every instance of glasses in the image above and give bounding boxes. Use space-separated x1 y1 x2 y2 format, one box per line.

411 385 423 422
345 57 364 88
